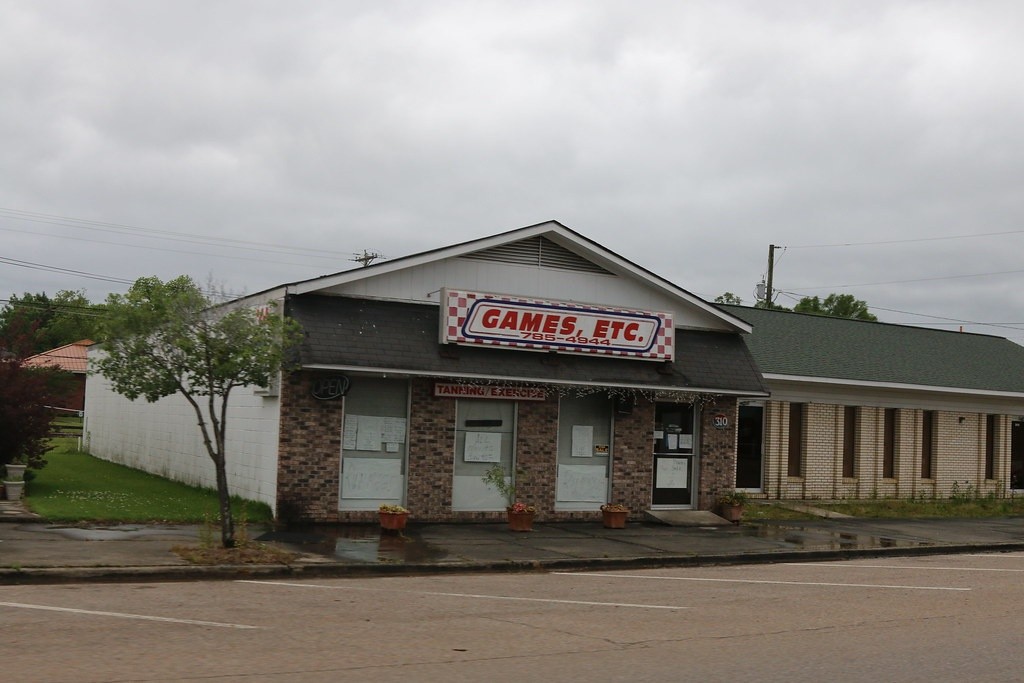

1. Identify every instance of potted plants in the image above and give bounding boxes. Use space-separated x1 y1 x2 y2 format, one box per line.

379 504 409 529
602 506 629 528
716 489 753 521
2 457 27 501
481 464 535 531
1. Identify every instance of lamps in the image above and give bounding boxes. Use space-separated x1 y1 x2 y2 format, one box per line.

959 417 965 423
1011 421 1019 426
